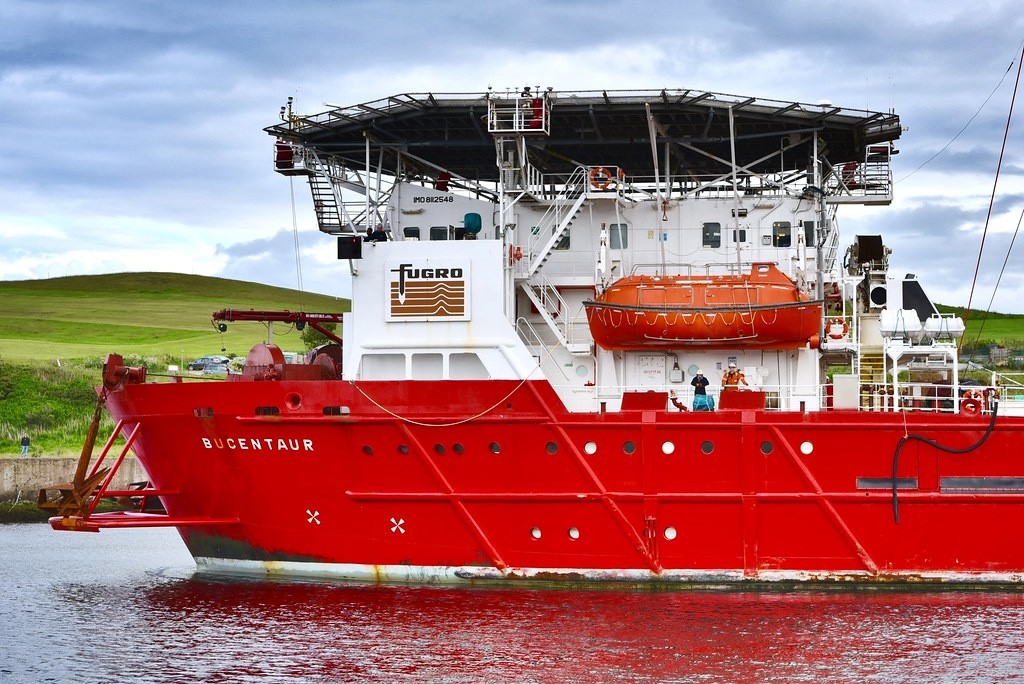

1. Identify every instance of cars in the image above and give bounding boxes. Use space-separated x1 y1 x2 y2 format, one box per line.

189 354 246 375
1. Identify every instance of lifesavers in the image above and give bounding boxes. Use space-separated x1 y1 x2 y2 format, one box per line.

590 168 612 189
963 390 986 413
826 317 847 339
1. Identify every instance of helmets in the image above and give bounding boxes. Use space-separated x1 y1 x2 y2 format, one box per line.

697 370 703 375
729 363 736 367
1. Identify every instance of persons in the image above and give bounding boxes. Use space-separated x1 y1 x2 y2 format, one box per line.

363 222 387 242
19 433 30 459
722 363 748 393
691 369 709 396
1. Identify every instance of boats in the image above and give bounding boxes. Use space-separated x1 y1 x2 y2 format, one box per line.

36 84 1024 610
581 262 826 352
